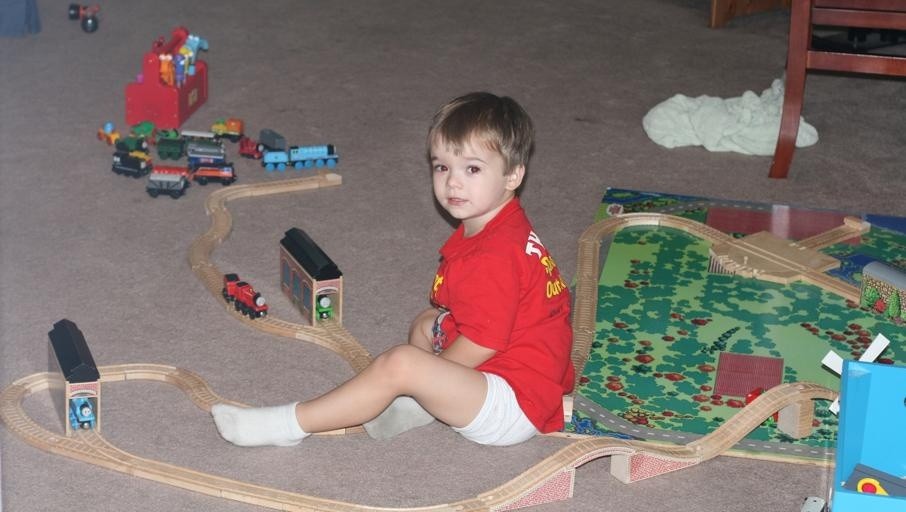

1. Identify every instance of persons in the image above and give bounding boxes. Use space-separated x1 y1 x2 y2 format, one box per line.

211 90 575 450
